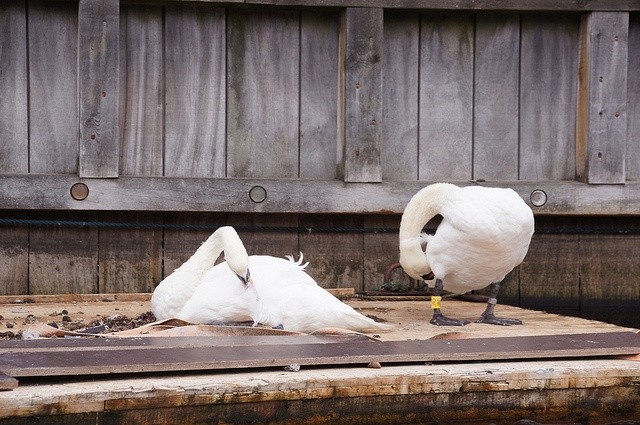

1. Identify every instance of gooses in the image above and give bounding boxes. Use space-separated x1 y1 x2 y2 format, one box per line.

151 225 395 334
398 183 535 325
